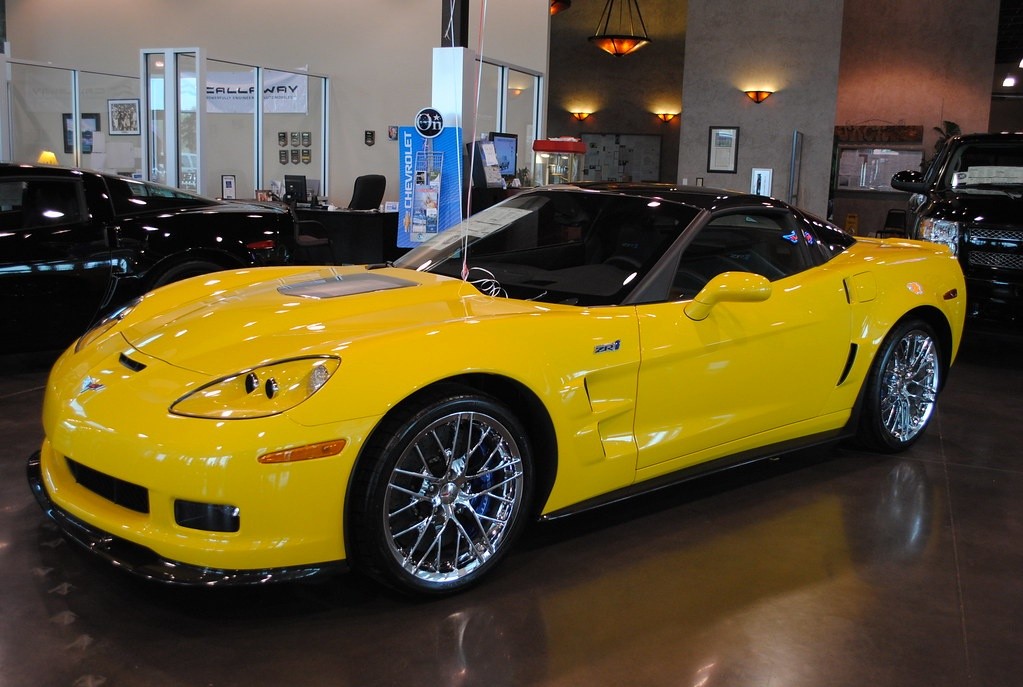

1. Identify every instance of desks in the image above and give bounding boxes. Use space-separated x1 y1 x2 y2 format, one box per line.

295 208 413 265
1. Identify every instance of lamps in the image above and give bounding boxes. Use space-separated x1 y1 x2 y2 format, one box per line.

36 149 59 165
570 113 593 123
550 0 571 16
588 0 652 59
745 91 772 104
655 114 677 128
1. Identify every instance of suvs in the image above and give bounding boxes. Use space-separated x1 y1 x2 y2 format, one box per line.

889 131 1023 351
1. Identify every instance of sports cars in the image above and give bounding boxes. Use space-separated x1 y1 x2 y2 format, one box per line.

25 180 971 600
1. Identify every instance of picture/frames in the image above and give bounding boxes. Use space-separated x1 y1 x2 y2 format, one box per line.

707 126 740 174
62 113 101 154
107 99 141 136
222 175 236 200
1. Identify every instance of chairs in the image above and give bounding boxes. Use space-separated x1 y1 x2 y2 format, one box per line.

296 221 337 266
876 209 907 239
348 175 386 210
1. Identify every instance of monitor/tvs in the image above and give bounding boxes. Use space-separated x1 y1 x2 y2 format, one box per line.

284 174 308 204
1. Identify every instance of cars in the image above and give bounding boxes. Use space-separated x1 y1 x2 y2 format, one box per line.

0 152 306 370
